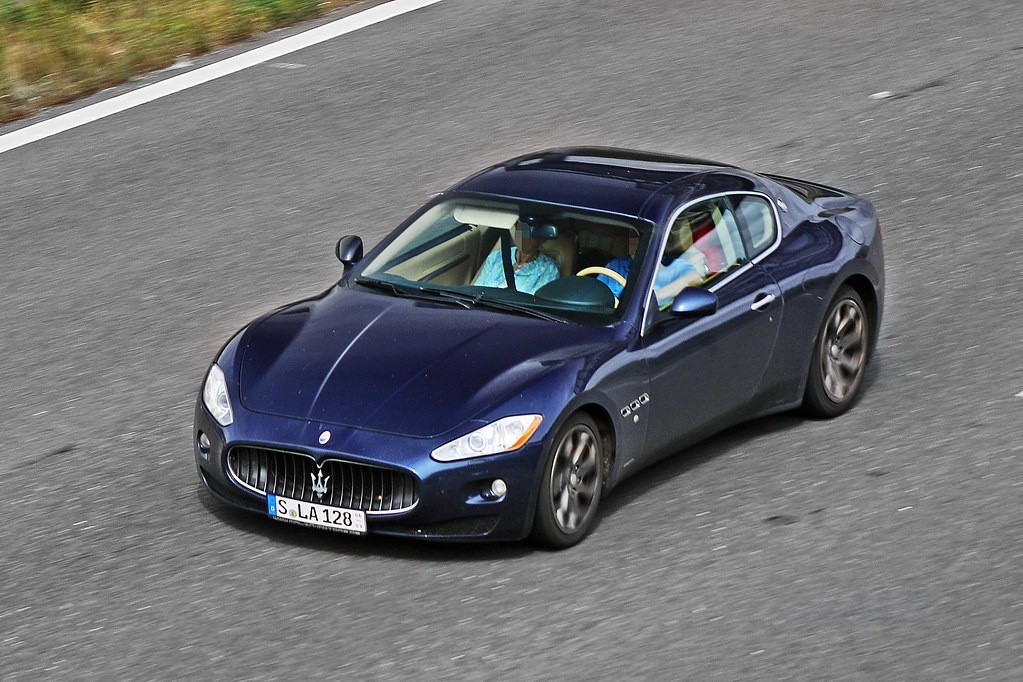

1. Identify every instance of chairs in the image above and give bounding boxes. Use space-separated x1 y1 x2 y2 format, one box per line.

468 222 576 287
670 219 707 280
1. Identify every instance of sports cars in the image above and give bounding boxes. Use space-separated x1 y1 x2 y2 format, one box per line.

193 146 885 549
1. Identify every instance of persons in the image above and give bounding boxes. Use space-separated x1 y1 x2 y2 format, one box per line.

597 232 706 307
473 226 559 296
